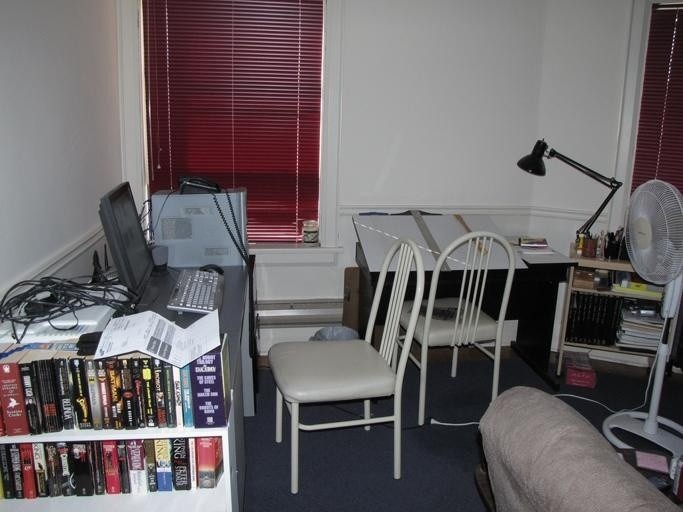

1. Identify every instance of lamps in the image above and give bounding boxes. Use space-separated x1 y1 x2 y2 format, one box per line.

516 137 624 238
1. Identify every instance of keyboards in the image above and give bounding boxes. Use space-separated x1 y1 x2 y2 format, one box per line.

166 268 225 315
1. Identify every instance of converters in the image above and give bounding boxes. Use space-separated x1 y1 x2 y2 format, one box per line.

25 295 58 318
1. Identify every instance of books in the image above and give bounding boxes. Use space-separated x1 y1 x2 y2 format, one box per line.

1 334 232 500
614 308 664 358
519 237 554 255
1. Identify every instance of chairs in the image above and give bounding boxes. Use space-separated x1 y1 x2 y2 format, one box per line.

265 235 424 496
397 231 516 425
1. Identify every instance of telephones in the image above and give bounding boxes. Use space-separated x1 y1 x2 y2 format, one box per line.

178 176 220 194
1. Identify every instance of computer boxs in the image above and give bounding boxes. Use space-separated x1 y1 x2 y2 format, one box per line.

151 186 250 268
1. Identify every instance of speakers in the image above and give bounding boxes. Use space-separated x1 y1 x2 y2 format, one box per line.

149 245 168 273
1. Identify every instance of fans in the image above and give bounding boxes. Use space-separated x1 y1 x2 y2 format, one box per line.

598 177 683 458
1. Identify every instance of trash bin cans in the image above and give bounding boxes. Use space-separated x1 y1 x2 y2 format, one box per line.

315 326 357 340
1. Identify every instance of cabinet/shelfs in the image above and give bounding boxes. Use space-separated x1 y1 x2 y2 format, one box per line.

555 252 683 381
0 400 241 511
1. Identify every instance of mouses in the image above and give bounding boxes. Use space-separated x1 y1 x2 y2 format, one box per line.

200 263 224 274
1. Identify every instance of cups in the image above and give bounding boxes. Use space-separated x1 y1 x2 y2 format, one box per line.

582 239 597 258
604 241 620 259
150 245 168 276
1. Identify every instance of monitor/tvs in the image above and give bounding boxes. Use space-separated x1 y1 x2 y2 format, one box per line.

98 181 156 297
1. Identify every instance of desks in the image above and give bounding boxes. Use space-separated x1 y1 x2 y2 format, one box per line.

136 256 263 512
351 211 577 391
